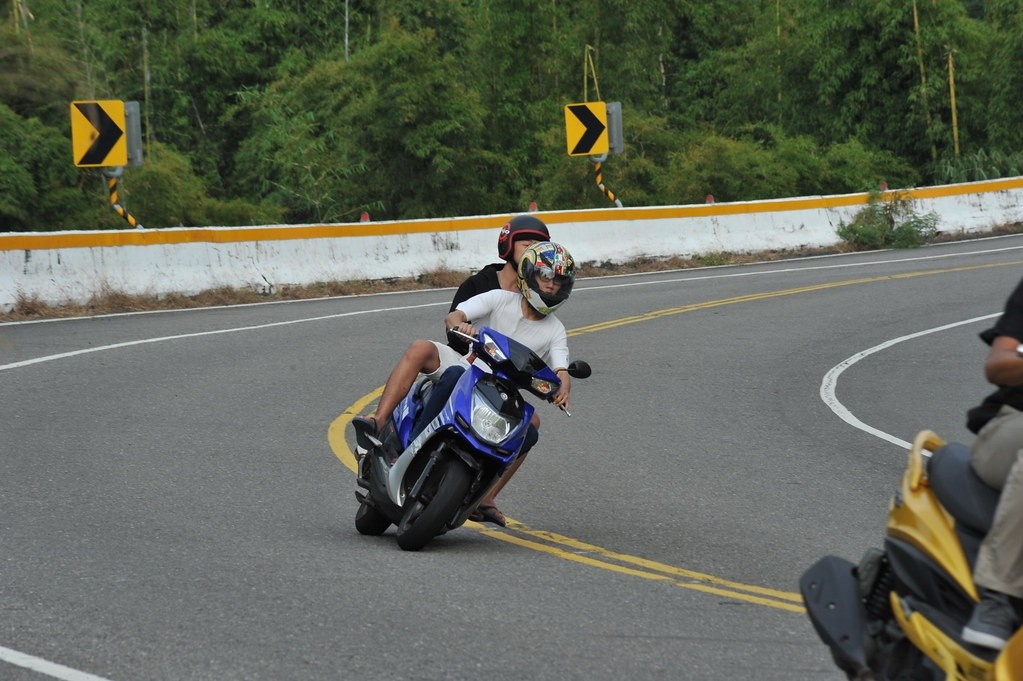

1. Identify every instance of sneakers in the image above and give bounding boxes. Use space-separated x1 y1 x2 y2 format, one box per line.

959 592 1021 652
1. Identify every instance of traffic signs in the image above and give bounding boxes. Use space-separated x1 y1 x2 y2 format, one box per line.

71 100 127 167
564 101 611 156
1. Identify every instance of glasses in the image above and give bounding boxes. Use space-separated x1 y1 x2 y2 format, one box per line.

537 271 565 285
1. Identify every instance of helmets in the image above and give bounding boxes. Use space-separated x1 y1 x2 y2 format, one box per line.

516 240 576 316
498 216 551 263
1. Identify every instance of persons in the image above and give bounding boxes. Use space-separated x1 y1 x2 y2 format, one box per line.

962 277 1023 650
351 242 575 528
446 214 552 355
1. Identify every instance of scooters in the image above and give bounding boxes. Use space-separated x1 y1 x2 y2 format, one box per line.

354 325 592 552
799 429 1023 681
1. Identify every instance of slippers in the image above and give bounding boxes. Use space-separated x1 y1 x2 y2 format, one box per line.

352 416 377 450
478 502 506 528
